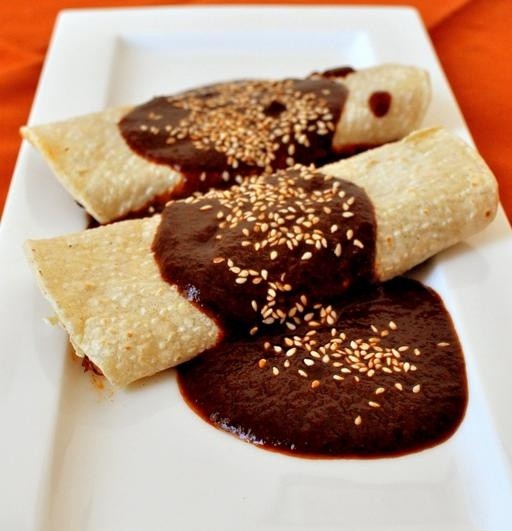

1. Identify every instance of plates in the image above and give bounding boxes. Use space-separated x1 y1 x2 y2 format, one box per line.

3 8 512 531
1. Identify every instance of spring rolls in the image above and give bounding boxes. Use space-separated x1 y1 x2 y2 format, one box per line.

22 126 500 391
18 62 431 224
